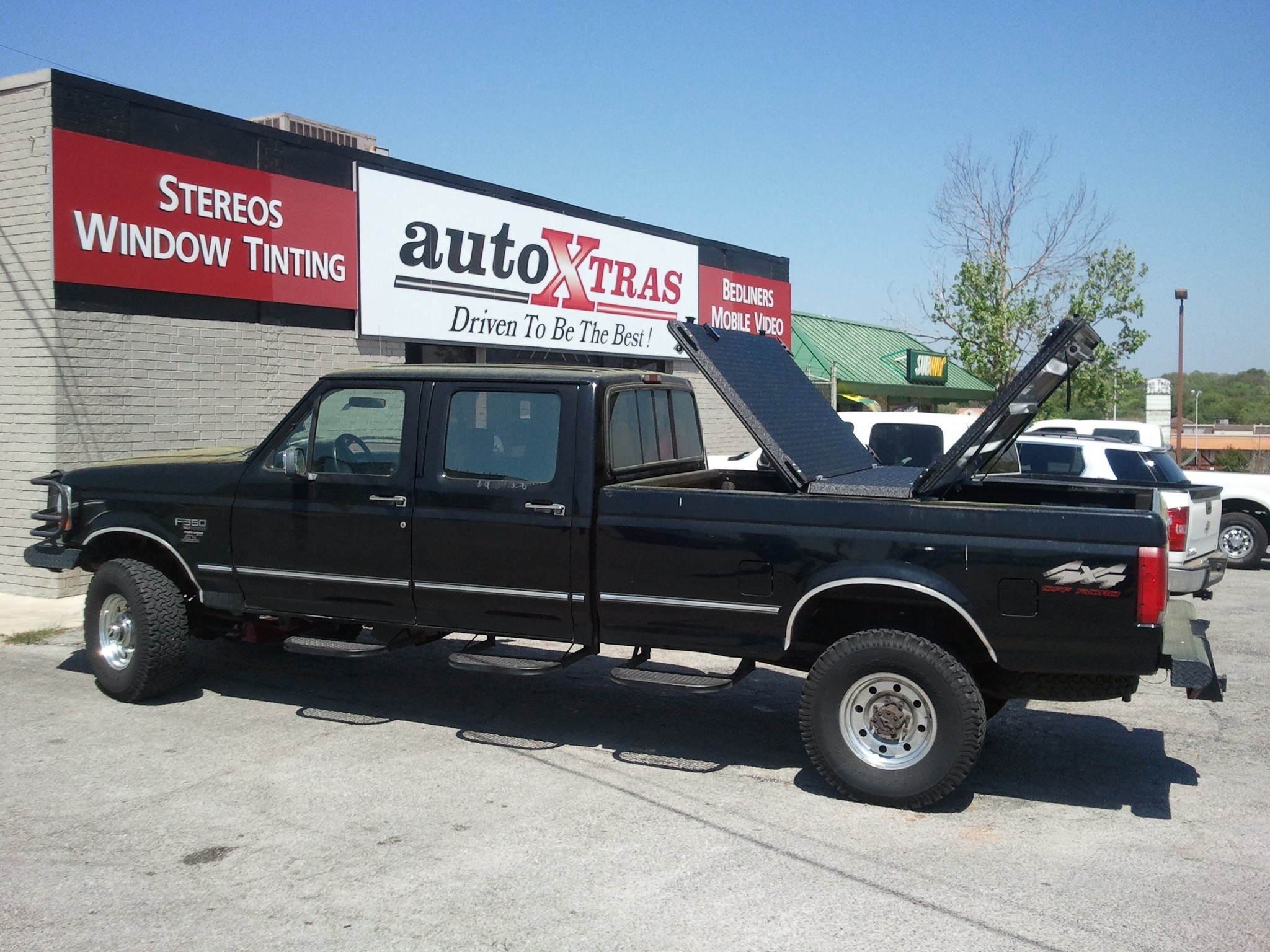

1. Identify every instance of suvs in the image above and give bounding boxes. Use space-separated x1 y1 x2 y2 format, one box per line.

959 431 1190 487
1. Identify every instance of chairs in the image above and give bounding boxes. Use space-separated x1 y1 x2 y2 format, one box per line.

453 428 494 473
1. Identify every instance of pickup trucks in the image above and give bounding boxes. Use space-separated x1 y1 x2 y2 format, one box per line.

703 413 1229 595
23 315 1227 810
1022 417 1270 571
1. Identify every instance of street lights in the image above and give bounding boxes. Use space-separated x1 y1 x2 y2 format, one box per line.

1174 289 1188 469
1189 389 1203 466
